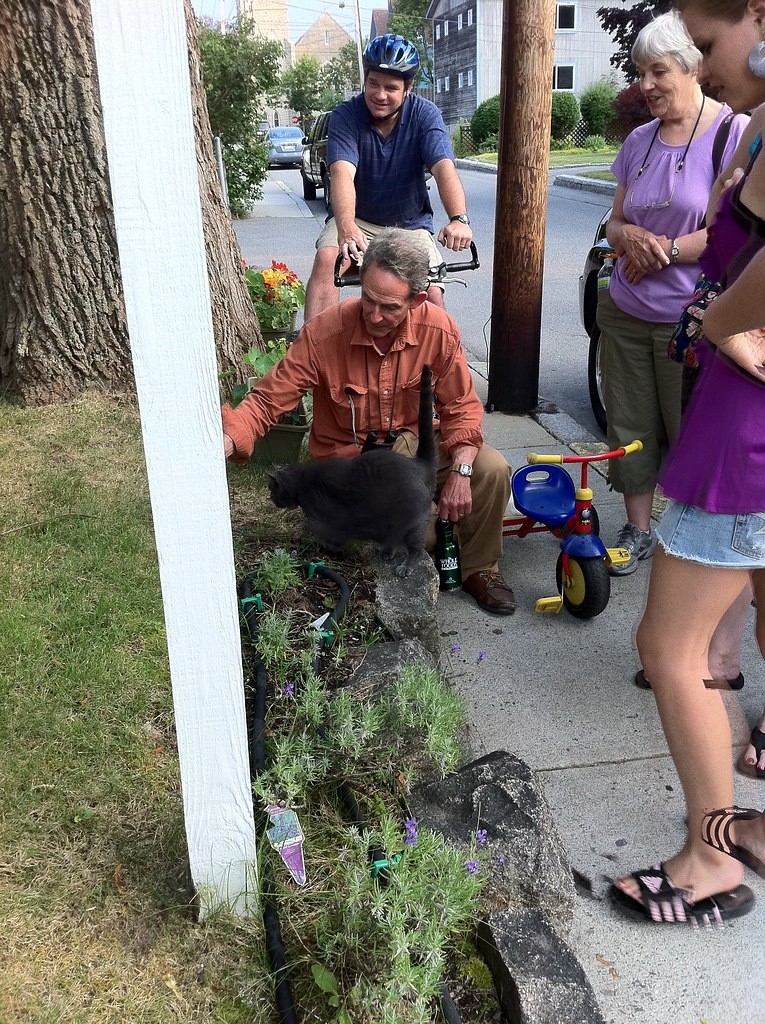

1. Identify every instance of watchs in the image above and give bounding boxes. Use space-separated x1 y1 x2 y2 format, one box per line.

671 238 679 264
450 214 470 224
448 464 474 477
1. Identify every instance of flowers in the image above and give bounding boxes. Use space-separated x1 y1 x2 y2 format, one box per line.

240 260 307 328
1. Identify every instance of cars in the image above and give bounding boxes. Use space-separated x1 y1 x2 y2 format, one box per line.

264 127 306 170
300 112 332 209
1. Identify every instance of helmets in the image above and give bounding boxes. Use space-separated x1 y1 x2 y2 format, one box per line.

363 30 423 78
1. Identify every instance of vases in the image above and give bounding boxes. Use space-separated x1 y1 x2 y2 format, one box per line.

279 305 297 335
261 327 288 351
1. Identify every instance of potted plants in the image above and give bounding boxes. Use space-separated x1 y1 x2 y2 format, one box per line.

219 349 312 463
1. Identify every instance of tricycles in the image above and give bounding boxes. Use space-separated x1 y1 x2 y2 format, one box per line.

502 439 644 620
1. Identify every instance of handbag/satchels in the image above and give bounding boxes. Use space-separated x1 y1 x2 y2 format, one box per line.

667 270 727 369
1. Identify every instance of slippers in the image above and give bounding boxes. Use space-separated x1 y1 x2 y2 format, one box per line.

683 793 765 881
608 860 753 928
633 662 744 693
737 720 765 780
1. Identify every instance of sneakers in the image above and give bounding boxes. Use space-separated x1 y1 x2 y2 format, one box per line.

607 519 659 577
460 568 515 617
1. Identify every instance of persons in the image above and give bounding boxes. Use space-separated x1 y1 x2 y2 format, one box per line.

596 11 752 574
635 566 765 781
216 228 516 614
610 2 765 928
304 34 474 326
